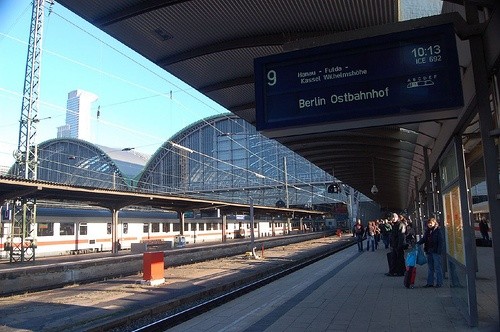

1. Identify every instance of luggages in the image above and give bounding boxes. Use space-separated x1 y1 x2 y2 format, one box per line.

387 246 405 273
404 266 416 288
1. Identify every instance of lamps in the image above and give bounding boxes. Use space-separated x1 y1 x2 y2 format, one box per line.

327 168 342 194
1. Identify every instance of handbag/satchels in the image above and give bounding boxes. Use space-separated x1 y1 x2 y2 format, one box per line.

416 245 426 264
406 246 416 265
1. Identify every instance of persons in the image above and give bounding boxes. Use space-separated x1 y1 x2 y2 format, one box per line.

352 215 415 252
384 213 407 276
416 218 443 288
479 219 490 240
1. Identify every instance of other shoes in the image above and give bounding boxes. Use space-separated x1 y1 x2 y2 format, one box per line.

436 284 440 287
386 272 392 276
423 284 432 288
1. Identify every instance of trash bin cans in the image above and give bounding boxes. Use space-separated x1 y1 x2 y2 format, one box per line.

173 235 185 248
